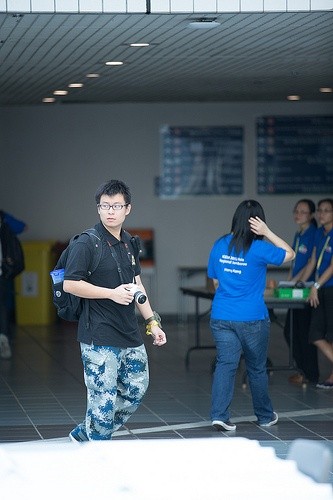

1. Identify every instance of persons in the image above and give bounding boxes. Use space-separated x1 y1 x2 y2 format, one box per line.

206 199 296 431
284 199 333 389
63 179 167 444
0 210 30 360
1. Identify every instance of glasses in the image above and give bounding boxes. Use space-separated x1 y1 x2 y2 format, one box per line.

97 202 128 211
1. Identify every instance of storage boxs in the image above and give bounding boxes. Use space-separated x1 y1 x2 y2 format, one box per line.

273 288 311 300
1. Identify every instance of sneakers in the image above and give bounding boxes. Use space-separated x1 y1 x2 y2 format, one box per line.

212 419 237 431
260 411 278 427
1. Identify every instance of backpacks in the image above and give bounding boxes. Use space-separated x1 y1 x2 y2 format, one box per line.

0 221 25 279
48 228 102 323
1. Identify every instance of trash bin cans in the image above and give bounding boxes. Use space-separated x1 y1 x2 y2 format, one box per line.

15 239 57 325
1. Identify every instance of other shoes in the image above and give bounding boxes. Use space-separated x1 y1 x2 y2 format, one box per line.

289 374 305 384
316 381 333 389
0 334 13 359
69 429 83 443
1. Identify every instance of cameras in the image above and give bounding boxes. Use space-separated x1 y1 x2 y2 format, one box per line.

126 283 146 304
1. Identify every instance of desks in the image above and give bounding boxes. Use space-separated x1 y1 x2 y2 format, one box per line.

178 266 291 329
180 286 309 390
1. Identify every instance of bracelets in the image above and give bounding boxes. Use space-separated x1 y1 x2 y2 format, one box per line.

314 281 321 289
143 311 162 335
